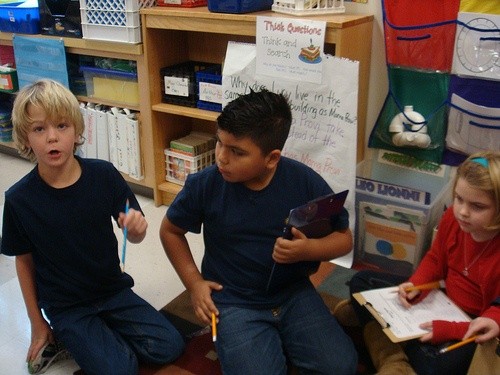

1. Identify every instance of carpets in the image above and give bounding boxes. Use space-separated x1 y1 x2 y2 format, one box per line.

73 262 477 375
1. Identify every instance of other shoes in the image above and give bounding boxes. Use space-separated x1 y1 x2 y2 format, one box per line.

28 338 72 375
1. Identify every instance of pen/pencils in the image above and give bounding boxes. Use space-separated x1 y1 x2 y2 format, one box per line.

441 334 480 354
121 200 129 271
211 312 216 341
387 282 439 294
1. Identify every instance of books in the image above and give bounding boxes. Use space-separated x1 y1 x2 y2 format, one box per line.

169 131 217 182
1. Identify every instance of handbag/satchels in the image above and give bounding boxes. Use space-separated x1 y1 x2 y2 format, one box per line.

345 270 404 304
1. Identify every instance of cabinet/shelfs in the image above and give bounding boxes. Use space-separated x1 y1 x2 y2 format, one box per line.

140 6 375 209
0 31 153 200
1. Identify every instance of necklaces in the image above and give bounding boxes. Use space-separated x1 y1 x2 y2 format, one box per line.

463 232 494 277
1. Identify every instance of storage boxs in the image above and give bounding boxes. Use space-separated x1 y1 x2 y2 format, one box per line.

353 160 453 274
0 0 346 190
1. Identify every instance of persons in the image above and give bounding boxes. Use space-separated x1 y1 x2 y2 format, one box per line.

1 78 185 375
159 87 359 375
333 150 500 375
